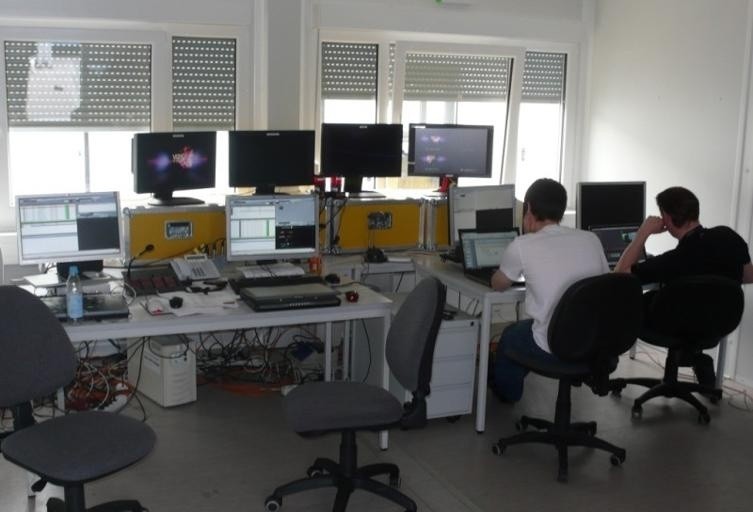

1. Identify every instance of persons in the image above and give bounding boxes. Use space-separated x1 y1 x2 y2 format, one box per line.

487 178 610 404
614 185 753 284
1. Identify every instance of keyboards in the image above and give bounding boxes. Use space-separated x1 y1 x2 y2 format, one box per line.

228 272 327 295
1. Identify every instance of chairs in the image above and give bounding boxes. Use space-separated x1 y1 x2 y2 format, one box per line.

1 283 159 512
493 272 645 474
259 272 447 510
604 265 746 420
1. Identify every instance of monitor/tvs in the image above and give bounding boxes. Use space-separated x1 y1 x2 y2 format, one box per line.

15 191 125 287
408 124 494 195
448 183 526 262
225 193 320 279
227 131 315 196
130 131 217 204
575 181 647 231
321 124 404 199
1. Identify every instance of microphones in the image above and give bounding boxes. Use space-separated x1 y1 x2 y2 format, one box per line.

128 243 155 284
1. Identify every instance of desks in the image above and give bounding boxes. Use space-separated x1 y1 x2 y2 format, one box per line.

411 246 737 439
19 233 396 448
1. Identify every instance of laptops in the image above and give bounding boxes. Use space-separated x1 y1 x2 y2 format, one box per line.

588 223 648 273
39 295 130 324
240 283 341 312
457 227 520 288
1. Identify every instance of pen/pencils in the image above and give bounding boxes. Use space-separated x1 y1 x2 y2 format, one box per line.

192 239 225 257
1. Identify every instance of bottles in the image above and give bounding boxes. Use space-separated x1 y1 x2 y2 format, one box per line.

64 266 83 325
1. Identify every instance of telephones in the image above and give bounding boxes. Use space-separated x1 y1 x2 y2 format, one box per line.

168 253 221 281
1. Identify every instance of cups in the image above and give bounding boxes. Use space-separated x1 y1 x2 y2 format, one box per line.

307 257 320 272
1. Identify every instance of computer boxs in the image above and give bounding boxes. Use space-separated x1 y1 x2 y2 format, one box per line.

127 334 197 408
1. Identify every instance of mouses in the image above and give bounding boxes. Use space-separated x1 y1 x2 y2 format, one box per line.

325 273 340 284
169 296 183 309
147 299 165 314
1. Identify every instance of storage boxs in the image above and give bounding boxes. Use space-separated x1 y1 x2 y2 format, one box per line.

124 206 230 267
421 190 451 252
318 191 426 254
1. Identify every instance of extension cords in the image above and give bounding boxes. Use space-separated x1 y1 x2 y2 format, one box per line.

249 374 293 392
90 394 128 414
204 355 259 369
